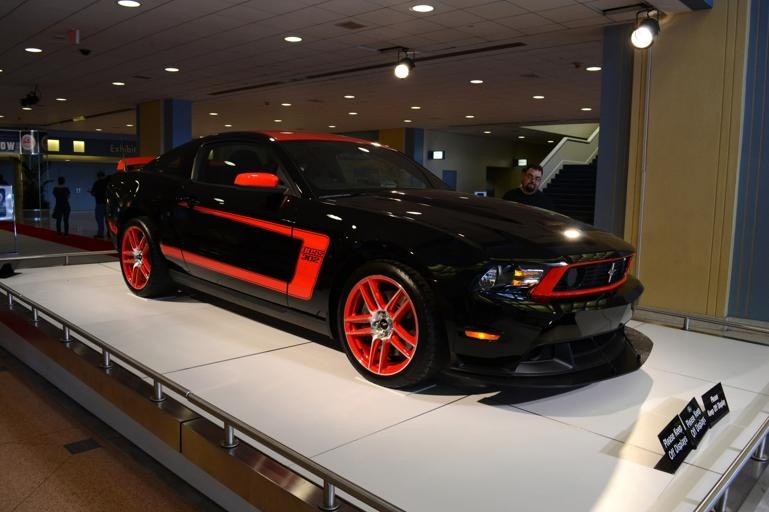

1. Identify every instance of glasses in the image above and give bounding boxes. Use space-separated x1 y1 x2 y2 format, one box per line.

524 174 542 182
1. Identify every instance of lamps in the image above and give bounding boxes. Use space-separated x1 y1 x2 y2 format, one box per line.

19 85 41 107
629 5 662 51
393 46 415 81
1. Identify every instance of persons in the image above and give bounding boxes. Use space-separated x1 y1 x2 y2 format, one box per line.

89 171 112 239
51 175 71 236
501 162 553 211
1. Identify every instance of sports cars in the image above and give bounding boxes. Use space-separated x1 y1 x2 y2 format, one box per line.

94 118 645 396
